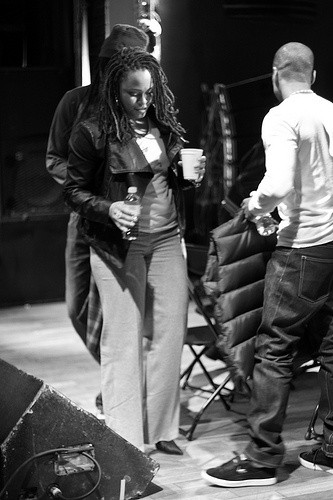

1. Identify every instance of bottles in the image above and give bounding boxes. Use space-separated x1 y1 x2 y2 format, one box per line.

249 191 276 236
122 187 141 240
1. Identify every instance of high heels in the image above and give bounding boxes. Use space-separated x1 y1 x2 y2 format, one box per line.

156 440 183 455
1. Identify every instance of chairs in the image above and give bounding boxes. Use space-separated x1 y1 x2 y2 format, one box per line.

181 230 323 442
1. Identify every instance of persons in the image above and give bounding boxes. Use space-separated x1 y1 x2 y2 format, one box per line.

64 49 206 455
46 24 152 409
201 42 333 488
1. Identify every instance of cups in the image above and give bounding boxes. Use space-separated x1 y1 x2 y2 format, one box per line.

181 148 203 179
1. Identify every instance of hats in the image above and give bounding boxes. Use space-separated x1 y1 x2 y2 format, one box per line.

98 24 150 58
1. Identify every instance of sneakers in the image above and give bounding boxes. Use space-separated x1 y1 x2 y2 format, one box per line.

297 447 333 474
201 454 278 488
